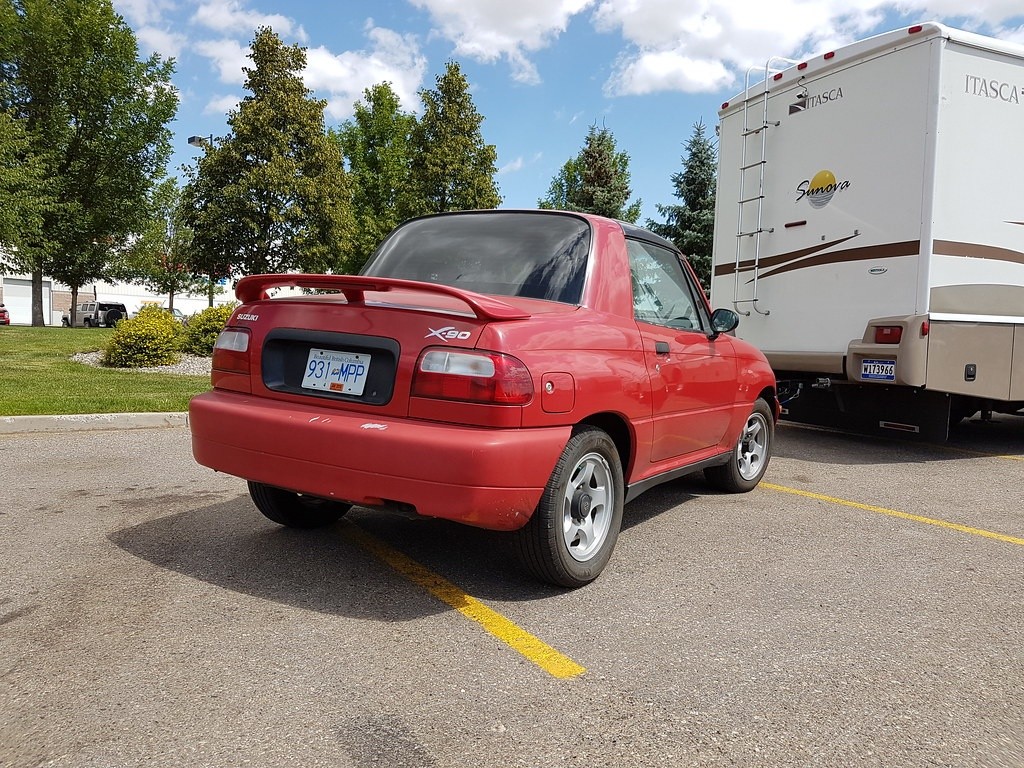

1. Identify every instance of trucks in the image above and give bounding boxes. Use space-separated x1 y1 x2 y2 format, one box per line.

709 21 1022 446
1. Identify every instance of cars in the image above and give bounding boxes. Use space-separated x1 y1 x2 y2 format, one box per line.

187 209 784 590
0 307 10 325
166 308 184 323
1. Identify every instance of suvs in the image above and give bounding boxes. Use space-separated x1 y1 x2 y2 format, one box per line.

62 301 128 328
